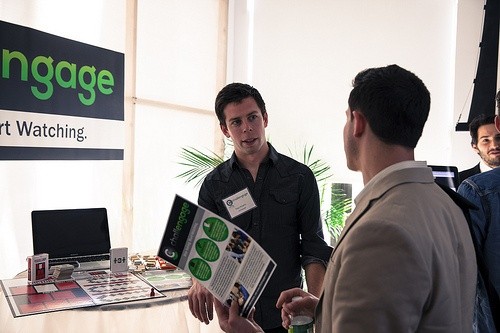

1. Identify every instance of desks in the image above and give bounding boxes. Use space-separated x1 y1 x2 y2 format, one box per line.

13 258 197 310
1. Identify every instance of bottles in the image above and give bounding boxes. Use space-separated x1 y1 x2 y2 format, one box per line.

287 296 314 333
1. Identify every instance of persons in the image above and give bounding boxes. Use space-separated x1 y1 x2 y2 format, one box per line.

186 83 333 333
213 64 476 333
456 90 500 333
